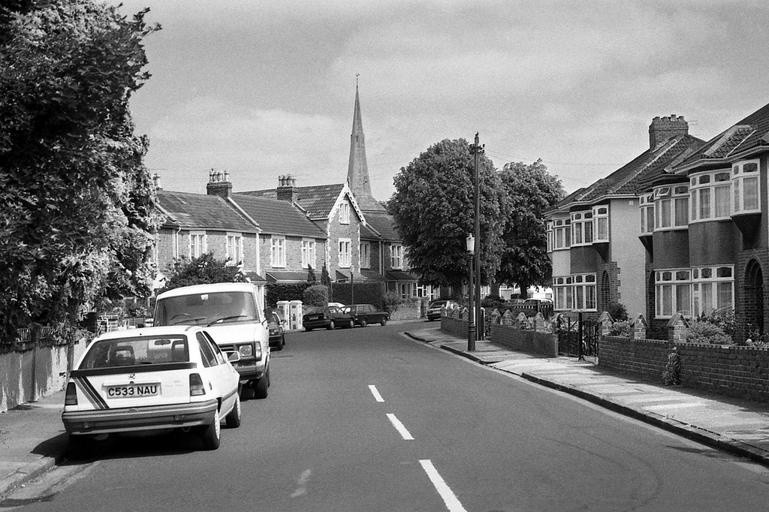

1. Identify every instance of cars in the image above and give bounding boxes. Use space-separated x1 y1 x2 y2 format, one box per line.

303 306 357 330
505 299 553 305
341 303 390 327
427 300 463 321
271 311 286 345
59 323 243 451
268 314 283 351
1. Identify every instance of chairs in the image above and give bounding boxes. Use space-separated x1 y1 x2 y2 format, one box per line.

172 340 188 361
109 346 135 365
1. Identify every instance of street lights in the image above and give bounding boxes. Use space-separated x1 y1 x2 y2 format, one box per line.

349 264 355 305
466 233 477 352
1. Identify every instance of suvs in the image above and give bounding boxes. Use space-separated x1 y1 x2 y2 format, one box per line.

150 283 273 400
